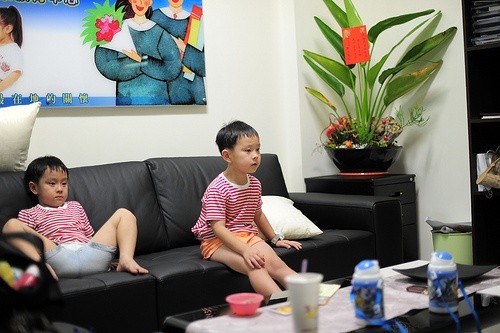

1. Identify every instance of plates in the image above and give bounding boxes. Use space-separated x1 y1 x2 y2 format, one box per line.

392 263 499 281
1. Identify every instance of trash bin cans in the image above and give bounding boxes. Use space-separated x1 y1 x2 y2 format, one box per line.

431 231 474 266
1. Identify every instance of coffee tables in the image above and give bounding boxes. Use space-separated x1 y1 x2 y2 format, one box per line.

159 253 500 333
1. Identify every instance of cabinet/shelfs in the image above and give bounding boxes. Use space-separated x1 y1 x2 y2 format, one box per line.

459 0 500 261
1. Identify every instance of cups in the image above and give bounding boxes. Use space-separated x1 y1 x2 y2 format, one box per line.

284 272 323 332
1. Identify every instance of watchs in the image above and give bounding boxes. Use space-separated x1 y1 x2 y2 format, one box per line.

271 235 283 245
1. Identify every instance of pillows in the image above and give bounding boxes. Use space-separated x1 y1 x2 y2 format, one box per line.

0 101 42 172
253 192 324 240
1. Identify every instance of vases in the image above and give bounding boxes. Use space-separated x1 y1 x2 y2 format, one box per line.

324 145 402 172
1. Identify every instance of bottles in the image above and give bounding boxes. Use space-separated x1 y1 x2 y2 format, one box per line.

427 252 459 312
350 259 385 325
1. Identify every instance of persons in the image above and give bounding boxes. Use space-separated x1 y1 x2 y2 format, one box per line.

191 121 304 301
2 156 149 280
0 6 27 97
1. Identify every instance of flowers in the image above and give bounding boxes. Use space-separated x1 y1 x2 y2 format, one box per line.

299 0 461 151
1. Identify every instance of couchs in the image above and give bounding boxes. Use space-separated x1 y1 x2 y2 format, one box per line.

0 151 402 333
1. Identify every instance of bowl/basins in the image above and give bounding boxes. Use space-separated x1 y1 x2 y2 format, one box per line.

225 293 264 316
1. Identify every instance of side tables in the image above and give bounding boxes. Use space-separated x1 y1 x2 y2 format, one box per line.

304 173 419 261
